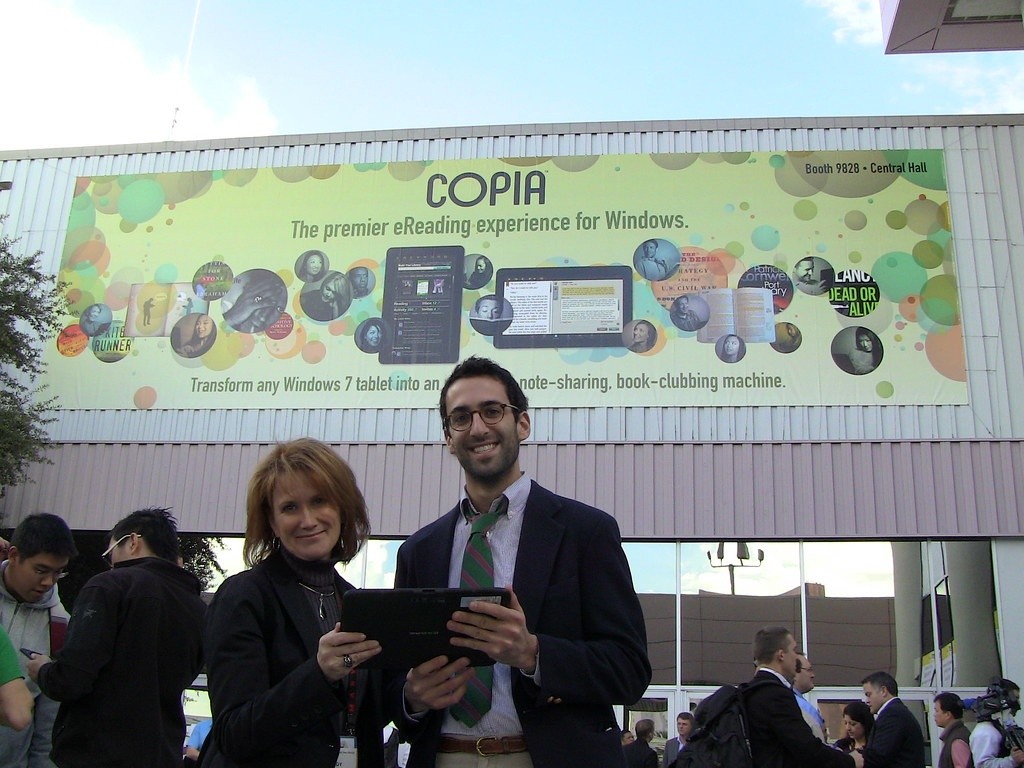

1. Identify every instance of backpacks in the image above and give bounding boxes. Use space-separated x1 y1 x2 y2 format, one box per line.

665 680 791 768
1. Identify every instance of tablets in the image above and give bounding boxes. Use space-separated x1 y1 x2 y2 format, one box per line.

20 648 57 664
339 587 509 667
493 266 632 347
379 245 465 362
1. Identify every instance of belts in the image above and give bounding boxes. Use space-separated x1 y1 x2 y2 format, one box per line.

437 736 527 756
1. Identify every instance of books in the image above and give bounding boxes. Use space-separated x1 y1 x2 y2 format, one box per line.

697 287 775 343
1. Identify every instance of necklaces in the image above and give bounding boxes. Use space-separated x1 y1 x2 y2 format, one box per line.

300 583 335 620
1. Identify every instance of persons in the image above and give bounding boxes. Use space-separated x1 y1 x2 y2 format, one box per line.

81 304 103 335
933 692 974 768
739 626 864 768
663 712 693 768
721 334 745 362
630 320 656 352
359 319 383 351
636 239 666 279
833 671 926 768
475 294 505 320
299 252 368 320
621 719 659 768
464 256 491 289
223 271 286 333
969 679 1024 768
849 328 874 374
672 295 707 330
0 508 211 768
197 438 381 768
178 313 216 356
794 257 827 294
380 356 653 768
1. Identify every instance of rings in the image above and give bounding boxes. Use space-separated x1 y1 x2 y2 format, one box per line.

254 295 263 303
343 654 353 668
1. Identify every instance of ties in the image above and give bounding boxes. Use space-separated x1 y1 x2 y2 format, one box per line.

449 510 501 728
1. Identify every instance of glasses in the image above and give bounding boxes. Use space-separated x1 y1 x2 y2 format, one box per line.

101 534 142 569
444 403 519 431
26 558 69 579
